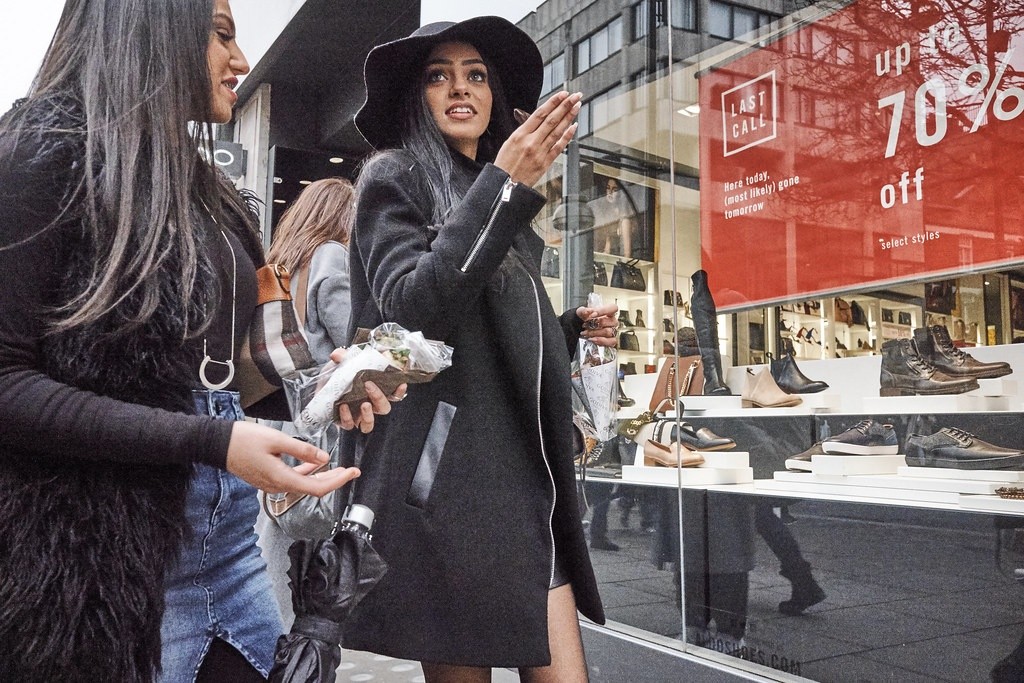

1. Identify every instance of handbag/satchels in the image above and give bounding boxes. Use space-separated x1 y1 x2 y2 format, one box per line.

833 296 870 332
619 329 657 375
540 247 646 293
881 308 911 324
263 413 339 538
926 285 978 344
661 289 684 354
650 356 704 413
230 267 318 421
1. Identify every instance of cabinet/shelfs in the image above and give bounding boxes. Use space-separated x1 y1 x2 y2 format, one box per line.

539 247 988 377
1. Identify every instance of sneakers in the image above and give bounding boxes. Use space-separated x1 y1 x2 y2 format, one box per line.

785 436 829 472
905 426 1024 471
823 419 901 454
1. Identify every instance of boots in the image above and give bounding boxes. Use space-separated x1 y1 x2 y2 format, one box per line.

914 326 1012 379
879 338 980 396
618 309 645 328
741 367 803 407
765 351 830 394
691 269 733 395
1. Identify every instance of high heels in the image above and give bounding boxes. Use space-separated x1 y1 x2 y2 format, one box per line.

779 320 822 345
670 426 737 451
642 440 704 467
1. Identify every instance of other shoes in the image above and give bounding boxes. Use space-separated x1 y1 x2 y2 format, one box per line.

684 568 826 652
591 508 654 551
781 515 799 525
857 338 873 349
834 337 847 349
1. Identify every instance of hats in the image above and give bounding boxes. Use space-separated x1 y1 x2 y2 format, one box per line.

355 15 545 154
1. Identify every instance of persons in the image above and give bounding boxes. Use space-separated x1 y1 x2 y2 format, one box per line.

600 178 643 261
578 476 1024 683
0 0 585 683
269 178 353 375
